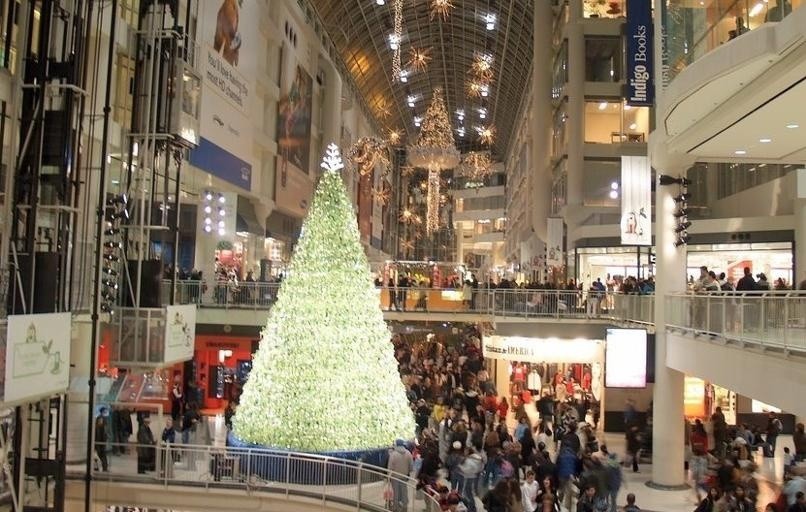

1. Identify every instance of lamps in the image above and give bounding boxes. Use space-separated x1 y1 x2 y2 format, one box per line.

658 172 692 249
99 191 131 316
202 189 227 237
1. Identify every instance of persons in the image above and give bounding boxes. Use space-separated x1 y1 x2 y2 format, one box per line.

726 16 751 43
92 262 806 511
764 0 793 24
276 65 310 175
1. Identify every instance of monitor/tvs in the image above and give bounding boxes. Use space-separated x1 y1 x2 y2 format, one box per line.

170 60 202 149
